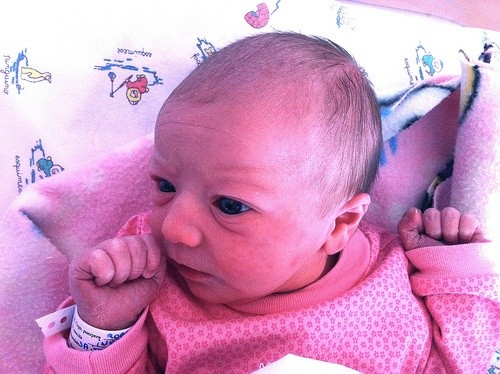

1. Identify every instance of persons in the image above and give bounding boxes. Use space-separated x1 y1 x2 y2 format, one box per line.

43 31 500 374
